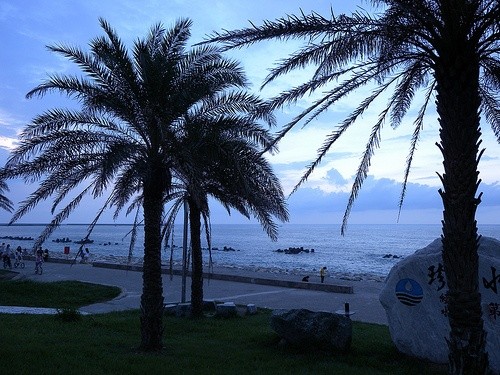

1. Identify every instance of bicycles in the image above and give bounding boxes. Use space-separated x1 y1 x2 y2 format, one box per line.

14 258 25 269
34 261 44 275
3 257 12 269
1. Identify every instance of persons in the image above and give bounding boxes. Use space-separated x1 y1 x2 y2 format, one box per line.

35 247 48 271
320 267 327 283
0 242 11 267
302 276 309 282
78 248 89 264
14 246 22 266
